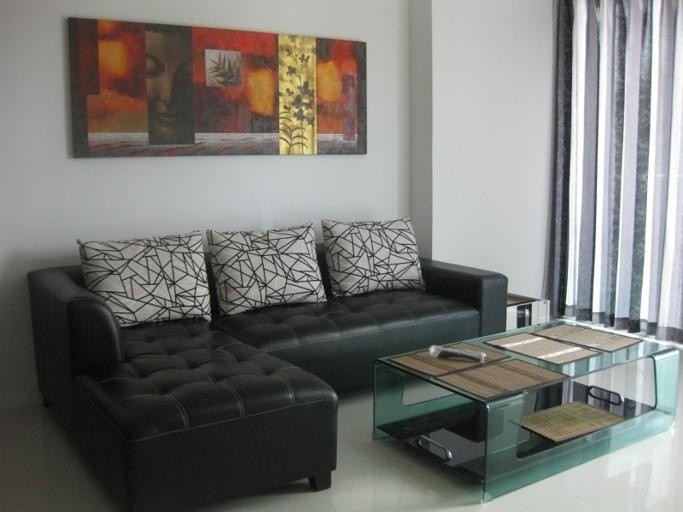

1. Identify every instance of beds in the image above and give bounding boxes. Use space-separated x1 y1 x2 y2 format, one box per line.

27 264 339 512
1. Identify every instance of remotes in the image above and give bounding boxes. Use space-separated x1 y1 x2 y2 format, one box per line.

438 348 484 362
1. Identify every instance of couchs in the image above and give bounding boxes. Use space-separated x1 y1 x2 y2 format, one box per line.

206 243 509 394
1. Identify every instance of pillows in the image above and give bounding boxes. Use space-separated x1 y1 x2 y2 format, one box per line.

206 222 329 316
321 216 427 298
76 229 212 328
